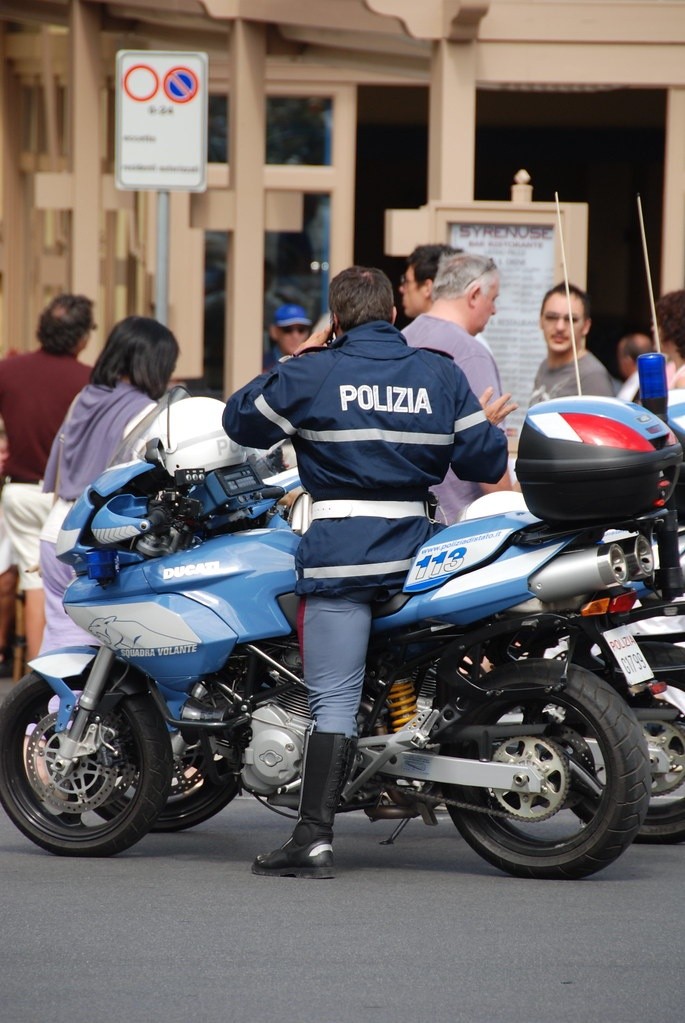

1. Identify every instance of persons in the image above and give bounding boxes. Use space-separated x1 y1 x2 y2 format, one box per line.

221 267 520 877
23 315 182 799
514 280 611 491
397 243 521 712
0 295 95 677
610 291 685 406
265 305 305 368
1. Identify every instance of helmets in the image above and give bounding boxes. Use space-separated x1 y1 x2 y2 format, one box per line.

143 397 248 480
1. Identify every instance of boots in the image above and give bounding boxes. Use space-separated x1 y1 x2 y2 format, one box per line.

251 729 359 879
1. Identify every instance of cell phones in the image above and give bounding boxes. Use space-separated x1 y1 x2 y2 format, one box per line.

327 313 336 346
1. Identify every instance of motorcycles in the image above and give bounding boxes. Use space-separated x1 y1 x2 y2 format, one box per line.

0 349 685 884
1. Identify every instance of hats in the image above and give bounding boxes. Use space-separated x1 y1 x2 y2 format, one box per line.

273 304 312 327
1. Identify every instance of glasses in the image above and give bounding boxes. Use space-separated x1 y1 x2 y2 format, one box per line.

544 311 579 325
400 273 415 285
281 325 305 334
